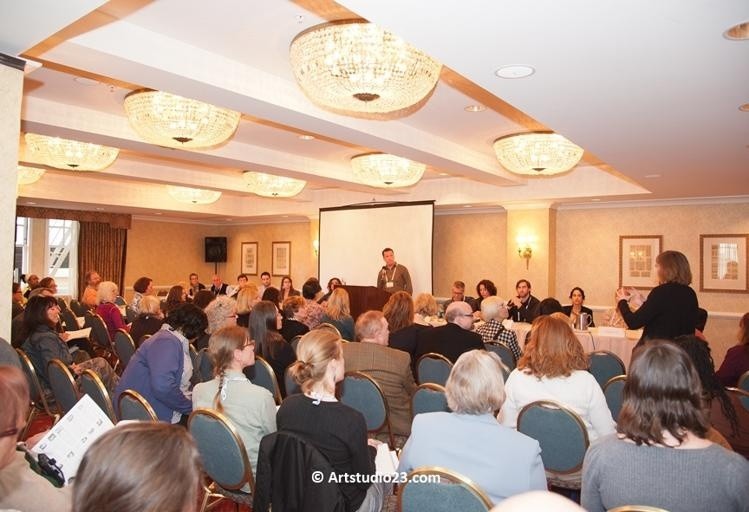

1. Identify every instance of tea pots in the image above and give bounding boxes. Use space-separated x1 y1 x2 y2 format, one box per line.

572 310 592 331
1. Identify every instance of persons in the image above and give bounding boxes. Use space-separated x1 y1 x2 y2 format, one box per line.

615 252 699 347
1 267 749 511
377 250 412 298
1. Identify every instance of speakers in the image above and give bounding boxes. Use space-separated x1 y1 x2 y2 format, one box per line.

205 237 227 262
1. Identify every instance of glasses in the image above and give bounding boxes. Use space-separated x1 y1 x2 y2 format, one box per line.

244 340 255 347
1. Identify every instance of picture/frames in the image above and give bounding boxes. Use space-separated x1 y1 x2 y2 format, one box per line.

618 234 664 290
271 240 292 277
241 241 258 276
698 234 749 294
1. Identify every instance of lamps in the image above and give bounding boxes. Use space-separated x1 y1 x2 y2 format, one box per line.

121 88 243 151
491 130 586 178
514 229 538 270
239 170 308 198
23 131 121 172
348 152 428 188
286 16 443 115
